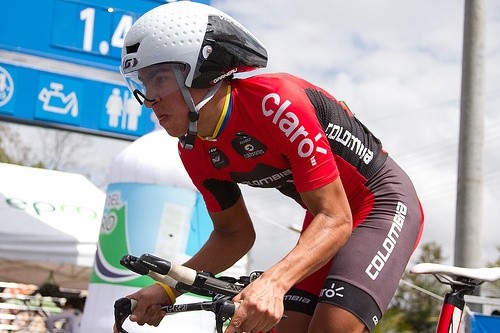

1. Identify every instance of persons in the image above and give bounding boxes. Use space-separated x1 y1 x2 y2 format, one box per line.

119 1 424 333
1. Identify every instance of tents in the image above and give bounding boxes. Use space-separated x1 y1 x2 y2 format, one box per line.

0 162 107 291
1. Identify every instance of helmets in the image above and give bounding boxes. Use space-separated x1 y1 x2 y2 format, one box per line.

119 1 268 105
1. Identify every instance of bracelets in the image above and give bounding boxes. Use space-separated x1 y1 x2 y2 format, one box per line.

155 281 176 304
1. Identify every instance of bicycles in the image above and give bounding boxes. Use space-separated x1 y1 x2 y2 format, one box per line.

114 253 500 333
0 296 85 333
